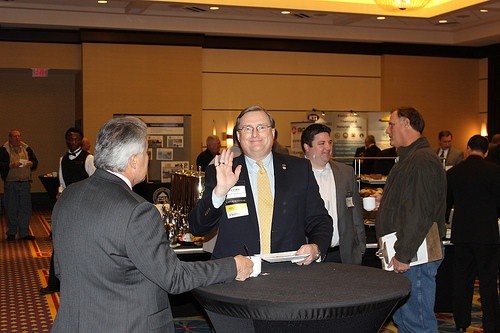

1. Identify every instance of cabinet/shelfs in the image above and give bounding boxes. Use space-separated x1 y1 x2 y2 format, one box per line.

333 157 449 228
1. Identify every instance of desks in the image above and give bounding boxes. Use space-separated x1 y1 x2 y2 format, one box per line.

191 261 412 333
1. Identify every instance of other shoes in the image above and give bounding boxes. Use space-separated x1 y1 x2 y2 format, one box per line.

20 235 36 240
456 327 466 333
39 286 60 294
7 234 16 242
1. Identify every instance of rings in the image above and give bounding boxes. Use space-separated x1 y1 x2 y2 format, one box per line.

215 162 220 167
220 162 224 164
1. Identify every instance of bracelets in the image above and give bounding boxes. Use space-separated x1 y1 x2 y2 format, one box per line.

313 244 320 260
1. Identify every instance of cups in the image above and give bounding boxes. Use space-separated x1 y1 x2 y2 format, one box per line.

363 197 375 211
246 256 261 278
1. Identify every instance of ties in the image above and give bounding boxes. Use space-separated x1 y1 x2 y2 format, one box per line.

440 151 444 163
256 160 274 255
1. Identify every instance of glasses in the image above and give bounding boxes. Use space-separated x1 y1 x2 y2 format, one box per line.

238 125 273 133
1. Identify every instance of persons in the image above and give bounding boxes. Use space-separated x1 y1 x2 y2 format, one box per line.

39 126 98 295
375 106 447 333
188 105 334 263
434 131 464 171
50 116 255 333
374 146 399 175
446 134 500 333
208 125 242 166
0 128 39 242
272 129 289 155
82 137 91 153
352 139 368 174
196 136 221 171
485 134 500 167
360 135 381 175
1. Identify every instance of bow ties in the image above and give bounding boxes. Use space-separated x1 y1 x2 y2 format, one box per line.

66 147 82 156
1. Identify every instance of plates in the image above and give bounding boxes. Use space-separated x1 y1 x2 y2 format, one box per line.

254 251 310 263
